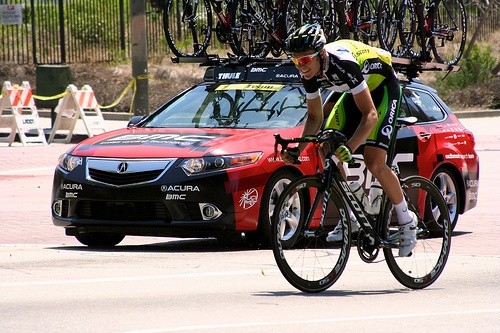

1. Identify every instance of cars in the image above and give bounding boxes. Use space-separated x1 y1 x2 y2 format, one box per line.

50 59 481 251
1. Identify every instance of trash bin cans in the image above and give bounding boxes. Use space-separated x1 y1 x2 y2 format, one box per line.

36 65 72 109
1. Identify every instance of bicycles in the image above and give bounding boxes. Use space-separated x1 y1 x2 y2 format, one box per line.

145 0 468 67
269 129 452 293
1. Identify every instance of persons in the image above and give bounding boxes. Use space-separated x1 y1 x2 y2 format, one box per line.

280 24 419 258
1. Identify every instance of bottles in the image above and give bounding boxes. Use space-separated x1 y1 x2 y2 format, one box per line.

368 179 384 214
349 181 371 214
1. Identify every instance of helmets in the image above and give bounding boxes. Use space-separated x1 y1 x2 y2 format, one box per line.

285 23 326 51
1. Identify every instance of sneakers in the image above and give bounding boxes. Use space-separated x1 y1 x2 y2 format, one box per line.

397 211 419 256
326 219 361 242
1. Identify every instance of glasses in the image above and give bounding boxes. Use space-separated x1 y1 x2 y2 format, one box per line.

290 50 321 66
